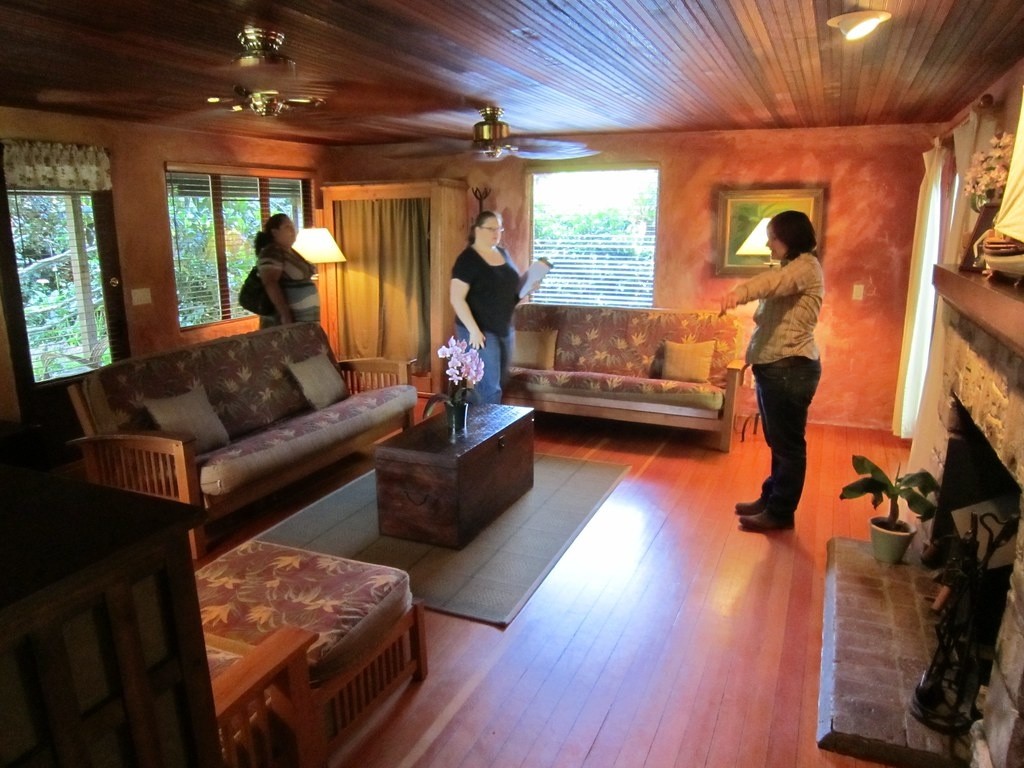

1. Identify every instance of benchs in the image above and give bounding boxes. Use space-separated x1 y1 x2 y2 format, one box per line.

503 298 759 453
68 317 418 558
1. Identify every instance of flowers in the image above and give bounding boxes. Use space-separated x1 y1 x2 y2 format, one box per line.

423 335 486 417
961 129 1014 213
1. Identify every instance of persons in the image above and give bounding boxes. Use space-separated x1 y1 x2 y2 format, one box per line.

450 210 540 406
719 210 825 531
254 213 320 331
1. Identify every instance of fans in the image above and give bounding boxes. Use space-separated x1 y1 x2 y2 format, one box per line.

387 104 601 166
158 26 330 129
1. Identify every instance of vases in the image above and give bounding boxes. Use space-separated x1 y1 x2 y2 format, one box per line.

444 401 470 445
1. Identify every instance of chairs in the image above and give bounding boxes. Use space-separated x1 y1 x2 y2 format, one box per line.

194 538 428 768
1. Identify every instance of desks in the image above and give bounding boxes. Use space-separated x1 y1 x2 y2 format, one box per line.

373 401 535 549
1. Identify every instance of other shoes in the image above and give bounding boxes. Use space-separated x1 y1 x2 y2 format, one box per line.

738 512 794 531
735 499 771 516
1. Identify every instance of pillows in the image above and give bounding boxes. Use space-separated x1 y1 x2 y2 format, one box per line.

514 329 557 369
289 353 347 411
143 385 234 455
663 339 715 380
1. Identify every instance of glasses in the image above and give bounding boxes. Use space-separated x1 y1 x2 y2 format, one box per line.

479 223 505 235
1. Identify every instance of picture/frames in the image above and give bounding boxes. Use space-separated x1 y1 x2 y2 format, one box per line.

713 188 826 275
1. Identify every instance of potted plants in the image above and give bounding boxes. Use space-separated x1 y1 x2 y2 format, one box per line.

839 453 940 566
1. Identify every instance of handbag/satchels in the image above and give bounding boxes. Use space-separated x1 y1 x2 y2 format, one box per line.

238 250 281 315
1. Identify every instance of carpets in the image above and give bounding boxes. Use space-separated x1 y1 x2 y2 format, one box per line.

252 445 630 626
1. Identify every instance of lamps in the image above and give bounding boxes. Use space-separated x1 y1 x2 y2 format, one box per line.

290 226 346 266
825 2 891 43
